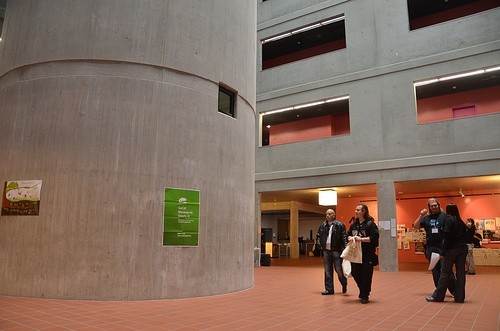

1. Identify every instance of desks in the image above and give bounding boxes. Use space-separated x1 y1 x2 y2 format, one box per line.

306 242 314 256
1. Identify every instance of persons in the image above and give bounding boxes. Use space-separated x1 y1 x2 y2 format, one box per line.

299 236 304 243
346 203 379 303
464 218 477 276
413 198 458 297
474 228 482 247
426 202 469 304
316 208 348 295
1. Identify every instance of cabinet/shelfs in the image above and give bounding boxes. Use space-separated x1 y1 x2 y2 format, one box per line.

265 242 279 257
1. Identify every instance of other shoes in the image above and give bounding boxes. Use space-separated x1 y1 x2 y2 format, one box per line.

466 273 475 275
359 293 362 298
426 297 444 302
466 271 468 273
454 297 464 302
361 299 368 303
449 289 464 302
321 291 334 295
343 286 346 292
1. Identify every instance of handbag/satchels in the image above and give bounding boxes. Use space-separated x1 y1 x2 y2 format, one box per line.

341 257 351 278
340 237 362 263
421 242 443 261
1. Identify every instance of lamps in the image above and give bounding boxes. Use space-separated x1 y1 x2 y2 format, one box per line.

318 189 338 206
